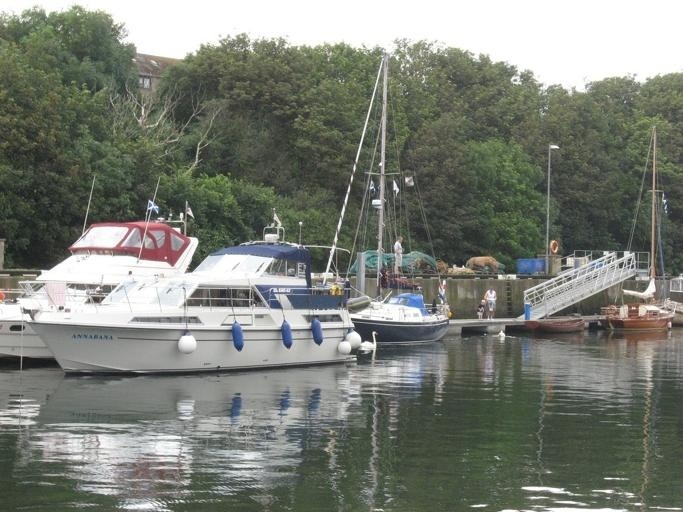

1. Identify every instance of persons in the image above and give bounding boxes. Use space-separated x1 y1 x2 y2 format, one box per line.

437 278 447 304
393 236 405 276
476 299 485 319
483 285 496 319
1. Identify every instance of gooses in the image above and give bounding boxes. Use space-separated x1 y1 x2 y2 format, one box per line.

357 331 379 350
499 330 505 337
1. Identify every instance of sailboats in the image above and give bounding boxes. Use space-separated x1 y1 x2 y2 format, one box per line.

321 55 452 349
598 126 676 329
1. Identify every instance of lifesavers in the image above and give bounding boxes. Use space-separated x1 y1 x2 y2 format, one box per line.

550 241 558 254
330 285 342 296
609 305 616 314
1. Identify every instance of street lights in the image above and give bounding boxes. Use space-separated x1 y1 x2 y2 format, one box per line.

544 144 561 276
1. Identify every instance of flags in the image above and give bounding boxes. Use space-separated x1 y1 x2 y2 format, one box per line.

404 176 415 187
661 192 668 215
549 145 559 149
369 180 376 193
146 200 159 215
392 181 399 197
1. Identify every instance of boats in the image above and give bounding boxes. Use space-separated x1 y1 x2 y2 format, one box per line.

0 174 200 367
658 271 682 327
33 360 362 424
19 176 377 374
523 312 584 333
